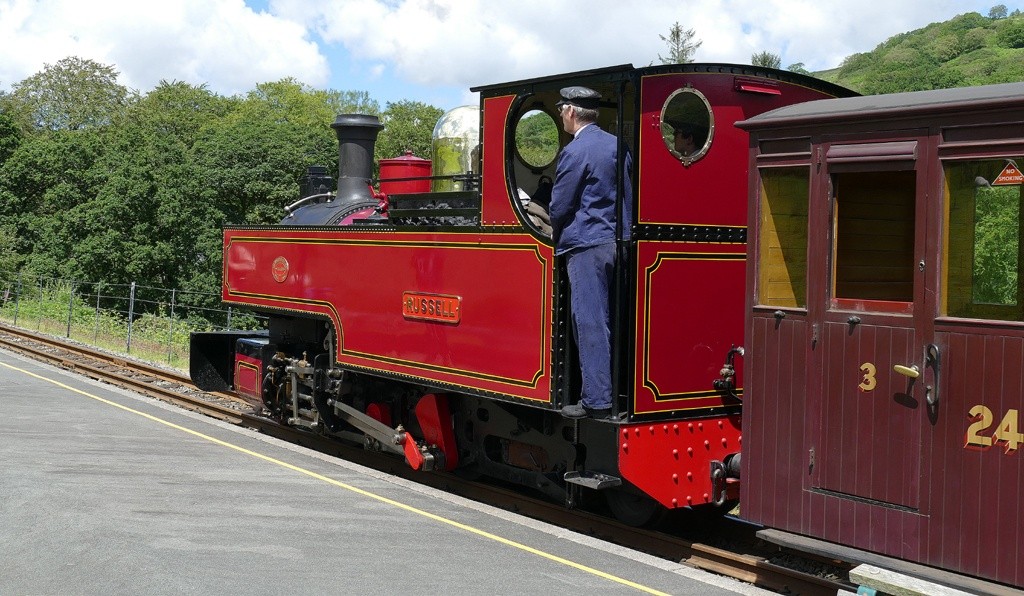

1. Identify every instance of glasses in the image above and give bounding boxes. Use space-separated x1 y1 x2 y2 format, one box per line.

557 105 567 113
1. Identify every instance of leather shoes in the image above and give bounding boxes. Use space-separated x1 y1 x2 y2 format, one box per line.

560 400 615 420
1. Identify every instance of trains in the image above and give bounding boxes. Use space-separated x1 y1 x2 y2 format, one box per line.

189 64 1023 596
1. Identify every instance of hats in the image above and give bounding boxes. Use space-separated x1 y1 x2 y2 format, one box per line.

555 86 603 109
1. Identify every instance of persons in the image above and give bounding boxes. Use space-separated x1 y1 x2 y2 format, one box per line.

667 104 709 156
549 87 631 420
